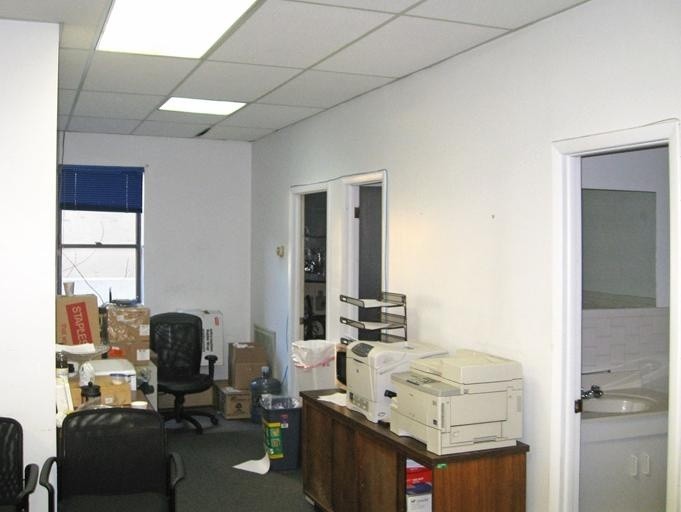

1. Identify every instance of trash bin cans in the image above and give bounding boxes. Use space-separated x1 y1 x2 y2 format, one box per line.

259 393 302 470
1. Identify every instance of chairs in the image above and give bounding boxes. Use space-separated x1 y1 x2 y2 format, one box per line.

0 417 39 512
39 410 184 512
150 312 219 434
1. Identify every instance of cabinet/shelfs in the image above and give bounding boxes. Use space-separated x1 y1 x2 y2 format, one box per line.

300 390 530 512
339 293 407 347
580 435 667 512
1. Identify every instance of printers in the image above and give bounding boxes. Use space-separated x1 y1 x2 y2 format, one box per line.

346 339 448 425
390 348 523 456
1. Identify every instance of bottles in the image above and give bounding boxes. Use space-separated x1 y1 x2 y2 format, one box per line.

57 351 70 375
81 381 102 408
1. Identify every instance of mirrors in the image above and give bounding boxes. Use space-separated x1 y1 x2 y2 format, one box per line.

582 189 657 309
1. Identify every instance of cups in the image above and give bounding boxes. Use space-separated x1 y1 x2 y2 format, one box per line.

64 281 75 295
130 401 147 409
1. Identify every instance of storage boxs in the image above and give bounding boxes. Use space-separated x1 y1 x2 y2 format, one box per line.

55 294 154 409
214 342 268 420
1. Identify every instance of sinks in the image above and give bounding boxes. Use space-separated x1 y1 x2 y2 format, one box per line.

581 398 648 414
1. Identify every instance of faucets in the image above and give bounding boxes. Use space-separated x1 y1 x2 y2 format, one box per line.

581 384 602 400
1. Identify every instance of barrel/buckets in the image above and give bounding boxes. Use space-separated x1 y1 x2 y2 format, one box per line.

250 366 280 425
